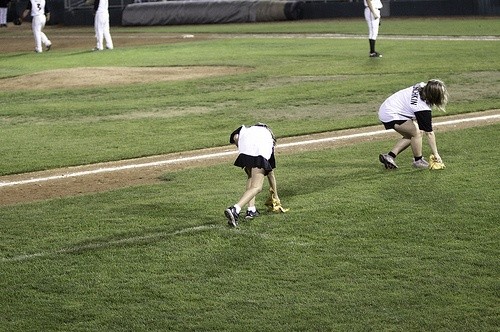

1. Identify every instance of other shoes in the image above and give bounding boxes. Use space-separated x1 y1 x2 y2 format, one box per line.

105 46 110 50
33 49 42 54
369 51 383 59
45 44 53 52
91 47 101 52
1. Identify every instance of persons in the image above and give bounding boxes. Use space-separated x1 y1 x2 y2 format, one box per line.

91 0 114 51
30 0 53 54
224 121 283 227
363 0 384 58
379 78 449 171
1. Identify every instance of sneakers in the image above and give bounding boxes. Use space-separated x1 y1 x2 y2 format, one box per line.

378 153 399 171
412 156 431 168
223 205 241 228
244 209 262 221
